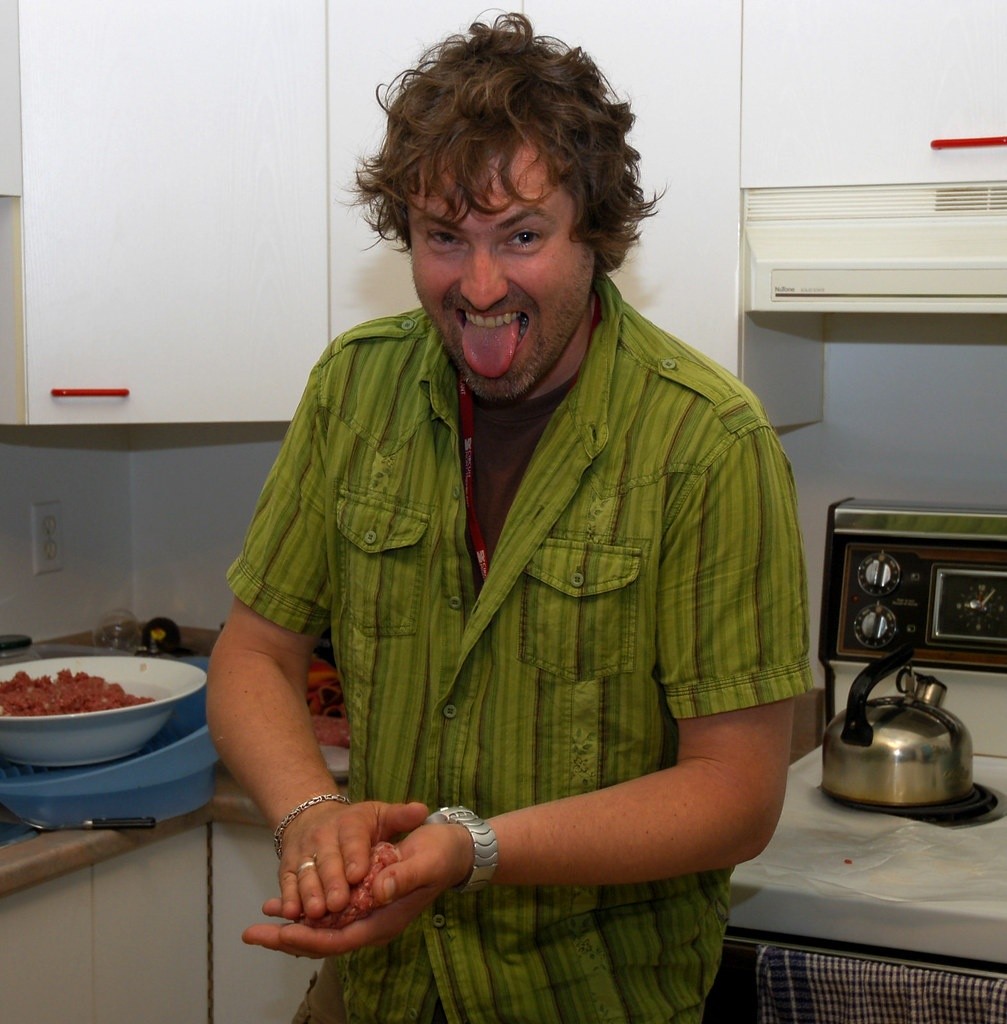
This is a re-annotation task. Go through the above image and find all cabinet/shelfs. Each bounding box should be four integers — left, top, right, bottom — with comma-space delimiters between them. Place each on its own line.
0, 820, 323, 1024
0, 0, 1007, 428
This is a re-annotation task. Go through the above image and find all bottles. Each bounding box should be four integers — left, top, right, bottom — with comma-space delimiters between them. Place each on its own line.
0, 634, 32, 665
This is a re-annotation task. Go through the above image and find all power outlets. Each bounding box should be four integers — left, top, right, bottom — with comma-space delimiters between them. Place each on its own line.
31, 500, 60, 575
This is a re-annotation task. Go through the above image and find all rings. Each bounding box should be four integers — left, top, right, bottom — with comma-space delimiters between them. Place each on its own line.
296, 863, 315, 874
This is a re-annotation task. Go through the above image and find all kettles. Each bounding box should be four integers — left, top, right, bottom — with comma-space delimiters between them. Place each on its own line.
823, 647, 973, 805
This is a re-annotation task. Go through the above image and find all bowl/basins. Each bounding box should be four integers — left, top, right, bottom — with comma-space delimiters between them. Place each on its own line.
0, 655, 207, 766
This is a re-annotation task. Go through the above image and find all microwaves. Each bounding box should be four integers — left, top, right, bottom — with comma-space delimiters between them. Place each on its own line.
819, 496, 1007, 676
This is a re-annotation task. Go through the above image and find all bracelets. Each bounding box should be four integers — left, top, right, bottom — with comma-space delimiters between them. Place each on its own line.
270, 793, 352, 861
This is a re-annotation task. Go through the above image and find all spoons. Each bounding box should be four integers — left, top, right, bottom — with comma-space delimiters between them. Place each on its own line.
20, 816, 156, 830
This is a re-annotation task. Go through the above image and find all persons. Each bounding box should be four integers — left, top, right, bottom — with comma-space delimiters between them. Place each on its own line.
205, 12, 810, 1024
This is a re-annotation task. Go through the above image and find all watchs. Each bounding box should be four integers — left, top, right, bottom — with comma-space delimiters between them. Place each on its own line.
422, 805, 498, 894
